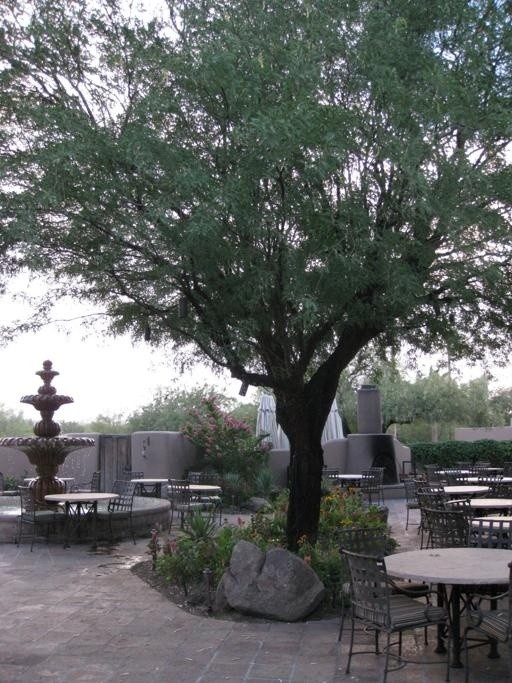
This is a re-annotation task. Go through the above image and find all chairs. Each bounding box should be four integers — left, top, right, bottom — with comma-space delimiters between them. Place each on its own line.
325, 457, 512, 530
337, 546, 451, 681
335, 528, 431, 646
15, 469, 225, 554
420, 507, 472, 547
462, 561, 512, 682
